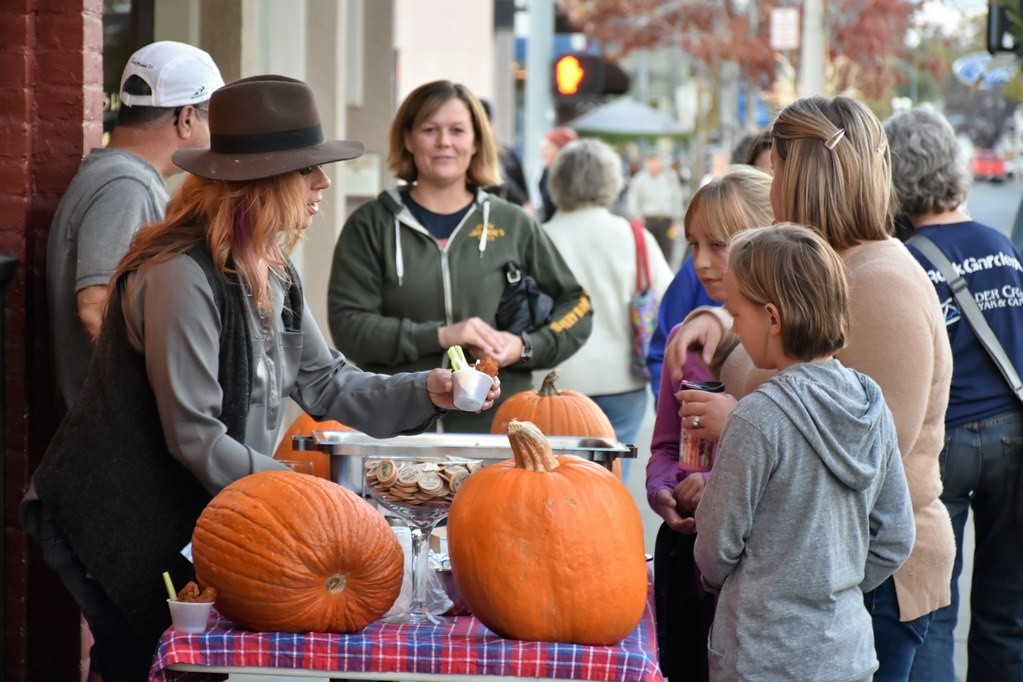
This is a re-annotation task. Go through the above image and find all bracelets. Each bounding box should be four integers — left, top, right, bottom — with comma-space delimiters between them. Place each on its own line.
519, 331, 532, 364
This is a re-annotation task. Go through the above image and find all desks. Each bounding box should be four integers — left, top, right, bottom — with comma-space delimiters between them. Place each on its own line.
148, 554, 669, 682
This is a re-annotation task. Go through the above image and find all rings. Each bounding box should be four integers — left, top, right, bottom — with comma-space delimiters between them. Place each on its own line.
693, 417, 699, 427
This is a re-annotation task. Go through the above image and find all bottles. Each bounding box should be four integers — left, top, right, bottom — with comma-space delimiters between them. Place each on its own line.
379, 517, 413, 618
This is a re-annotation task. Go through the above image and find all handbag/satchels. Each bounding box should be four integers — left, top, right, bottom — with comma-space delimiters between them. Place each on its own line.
495, 262, 553, 336
631, 216, 677, 377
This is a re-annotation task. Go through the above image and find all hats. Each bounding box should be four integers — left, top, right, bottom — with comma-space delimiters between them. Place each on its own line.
114, 41, 225, 105
170, 74, 365, 181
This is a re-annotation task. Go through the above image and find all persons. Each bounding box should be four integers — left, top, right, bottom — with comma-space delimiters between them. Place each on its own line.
540, 141, 676, 487
646, 170, 774, 682
883, 108, 1023, 682
693, 222, 916, 682
665, 96, 956, 682
19, 75, 501, 682
47, 40, 226, 682
327, 79, 595, 432
478, 98, 577, 220
646, 135, 773, 413
625, 152, 734, 266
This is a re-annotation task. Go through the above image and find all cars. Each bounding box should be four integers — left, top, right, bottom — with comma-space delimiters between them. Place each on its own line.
970, 149, 1005, 184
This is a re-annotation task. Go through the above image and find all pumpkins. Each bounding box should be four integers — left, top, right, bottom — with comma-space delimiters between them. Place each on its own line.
447, 418, 649, 647
491, 370, 622, 481
191, 471, 406, 634
271, 410, 358, 481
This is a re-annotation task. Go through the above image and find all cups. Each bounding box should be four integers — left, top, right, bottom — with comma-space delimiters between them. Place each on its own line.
166, 599, 215, 634
451, 369, 495, 413
678, 381, 726, 472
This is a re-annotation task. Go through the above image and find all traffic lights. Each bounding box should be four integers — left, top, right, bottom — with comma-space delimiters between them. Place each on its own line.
548, 52, 602, 100
